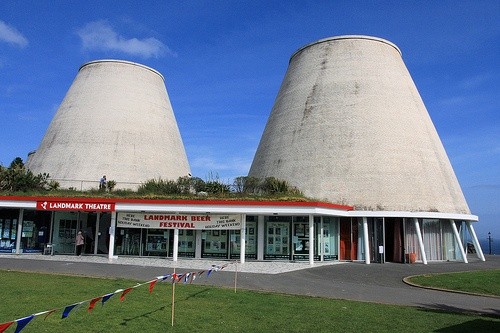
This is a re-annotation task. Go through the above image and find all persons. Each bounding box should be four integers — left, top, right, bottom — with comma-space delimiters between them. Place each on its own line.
76, 231, 84, 256
99, 176, 107, 191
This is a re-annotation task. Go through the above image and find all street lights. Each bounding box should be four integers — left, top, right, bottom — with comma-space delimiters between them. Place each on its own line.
488, 232, 493, 254
